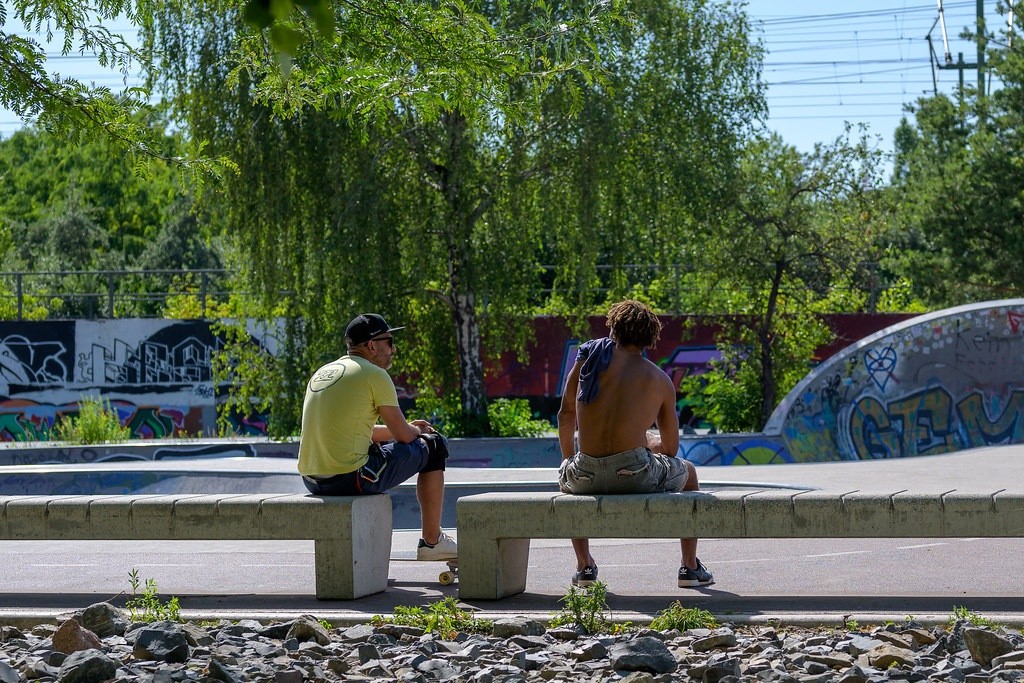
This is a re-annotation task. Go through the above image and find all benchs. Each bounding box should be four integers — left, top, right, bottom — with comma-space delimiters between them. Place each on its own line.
454, 484, 1024, 608
0, 488, 394, 606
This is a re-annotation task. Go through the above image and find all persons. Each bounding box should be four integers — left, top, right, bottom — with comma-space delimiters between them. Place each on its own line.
298, 313, 457, 561
557, 300, 714, 587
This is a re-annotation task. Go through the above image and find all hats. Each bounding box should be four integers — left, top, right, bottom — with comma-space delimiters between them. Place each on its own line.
345, 313, 406, 347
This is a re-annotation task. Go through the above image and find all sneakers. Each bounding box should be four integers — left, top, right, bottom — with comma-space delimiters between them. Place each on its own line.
416, 533, 457, 561
678, 557, 714, 587
572, 566, 598, 586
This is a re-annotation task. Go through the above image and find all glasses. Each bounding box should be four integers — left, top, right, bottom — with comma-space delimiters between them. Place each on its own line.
364, 336, 394, 348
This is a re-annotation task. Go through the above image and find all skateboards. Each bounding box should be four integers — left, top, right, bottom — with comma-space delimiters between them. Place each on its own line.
389, 556, 457, 586
